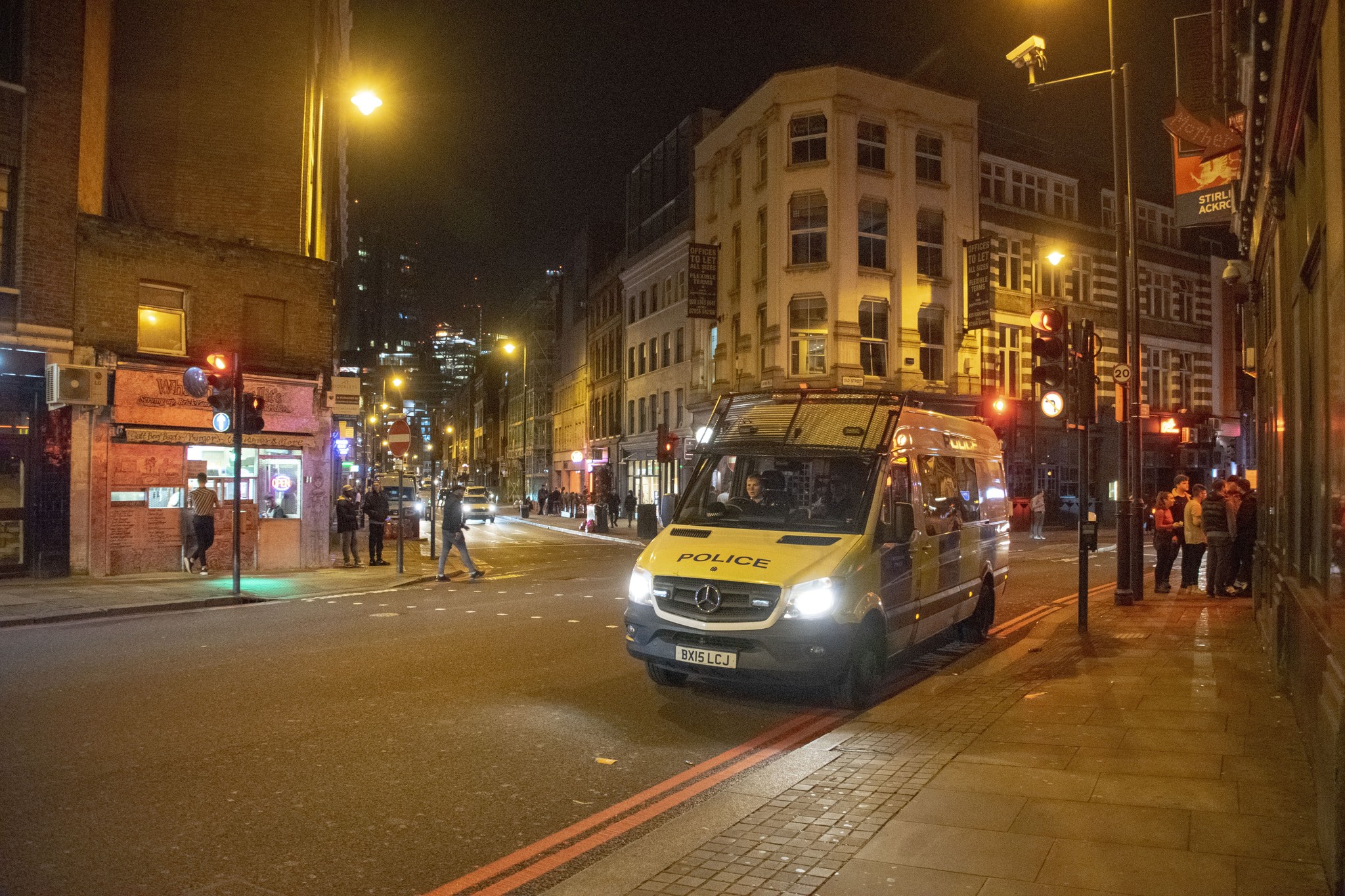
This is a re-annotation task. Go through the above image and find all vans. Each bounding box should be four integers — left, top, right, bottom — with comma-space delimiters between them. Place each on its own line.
624, 389, 1010, 711
374, 472, 432, 538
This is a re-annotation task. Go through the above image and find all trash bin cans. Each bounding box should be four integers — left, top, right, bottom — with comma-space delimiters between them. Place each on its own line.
520, 505, 530, 518
402, 506, 421, 538
636, 504, 658, 539
587, 502, 610, 532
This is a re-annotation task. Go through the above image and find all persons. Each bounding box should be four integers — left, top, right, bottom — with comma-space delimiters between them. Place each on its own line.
166, 473, 220, 576
435, 485, 484, 581
259, 493, 284, 518
336, 479, 390, 567
1029, 489, 1047, 540
708, 473, 848, 517
280, 481, 298, 515
519, 484, 636, 529
1146, 474, 1256, 597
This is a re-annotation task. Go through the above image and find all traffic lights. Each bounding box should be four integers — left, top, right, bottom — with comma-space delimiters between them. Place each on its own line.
244, 394, 264, 435
1030, 304, 1069, 434
663, 442, 673, 464
1067, 319, 1097, 425
205, 352, 235, 434
655, 423, 667, 464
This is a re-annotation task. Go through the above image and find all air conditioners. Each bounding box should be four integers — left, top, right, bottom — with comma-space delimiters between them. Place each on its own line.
46, 363, 108, 405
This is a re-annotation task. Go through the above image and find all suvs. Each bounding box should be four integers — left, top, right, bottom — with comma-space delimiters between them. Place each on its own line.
464, 486, 489, 499
462, 494, 495, 523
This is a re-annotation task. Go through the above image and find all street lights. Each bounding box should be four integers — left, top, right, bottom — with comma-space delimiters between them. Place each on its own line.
364, 393, 388, 486
382, 376, 401, 471
1028, 234, 1067, 513
504, 331, 526, 505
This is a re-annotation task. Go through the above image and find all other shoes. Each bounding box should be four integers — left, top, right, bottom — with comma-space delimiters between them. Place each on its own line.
548, 514, 560, 516
200, 568, 208, 575
184, 557, 194, 575
611, 526, 614, 528
343, 560, 354, 567
469, 570, 485, 580
369, 559, 377, 566
613, 521, 618, 527
354, 559, 369, 567
376, 559, 390, 566
1034, 535, 1046, 539
436, 574, 451, 582
1154, 581, 1238, 599
628, 524, 631, 527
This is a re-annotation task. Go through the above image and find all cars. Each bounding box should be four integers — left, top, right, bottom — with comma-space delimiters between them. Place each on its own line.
437, 487, 450, 500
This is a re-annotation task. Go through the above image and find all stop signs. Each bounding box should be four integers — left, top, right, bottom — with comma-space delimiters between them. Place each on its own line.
388, 420, 410, 457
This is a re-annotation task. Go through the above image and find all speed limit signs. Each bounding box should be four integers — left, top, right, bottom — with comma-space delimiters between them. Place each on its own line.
1112, 363, 1131, 383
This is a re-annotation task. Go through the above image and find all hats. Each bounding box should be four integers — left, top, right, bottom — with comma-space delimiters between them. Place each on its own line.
342, 484, 353, 492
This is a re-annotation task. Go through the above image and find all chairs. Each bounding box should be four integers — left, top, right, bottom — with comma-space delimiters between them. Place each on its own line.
761, 470, 793, 509
